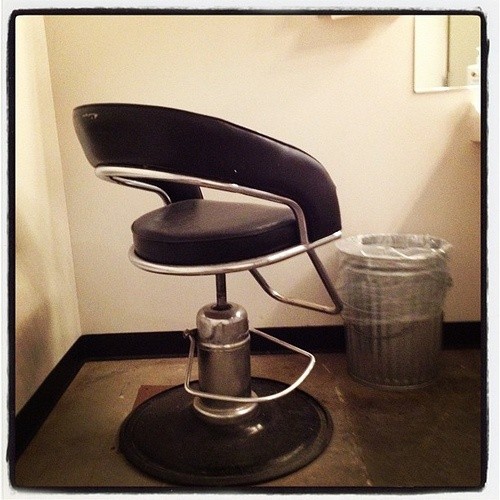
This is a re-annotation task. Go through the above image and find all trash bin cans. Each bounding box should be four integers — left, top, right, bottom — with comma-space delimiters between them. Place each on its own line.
335, 234, 453, 391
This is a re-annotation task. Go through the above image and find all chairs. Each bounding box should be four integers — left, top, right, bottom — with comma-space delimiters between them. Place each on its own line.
73, 103, 344, 487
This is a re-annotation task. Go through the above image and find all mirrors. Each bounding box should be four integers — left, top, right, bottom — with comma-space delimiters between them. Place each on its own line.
446, 16, 481, 86
